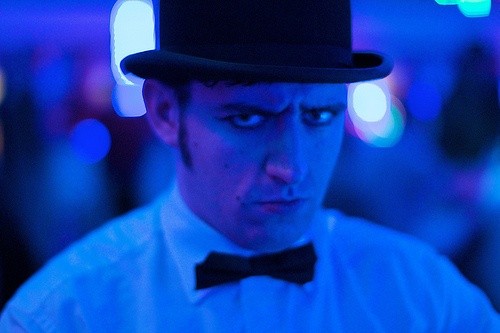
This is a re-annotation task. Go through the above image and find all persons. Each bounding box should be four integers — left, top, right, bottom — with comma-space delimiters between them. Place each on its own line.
1, 1, 500, 333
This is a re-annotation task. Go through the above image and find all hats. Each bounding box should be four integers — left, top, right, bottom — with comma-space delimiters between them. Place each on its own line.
119, 0, 394, 82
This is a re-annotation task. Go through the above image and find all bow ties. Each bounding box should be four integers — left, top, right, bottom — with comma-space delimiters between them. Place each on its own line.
194, 240, 317, 290
